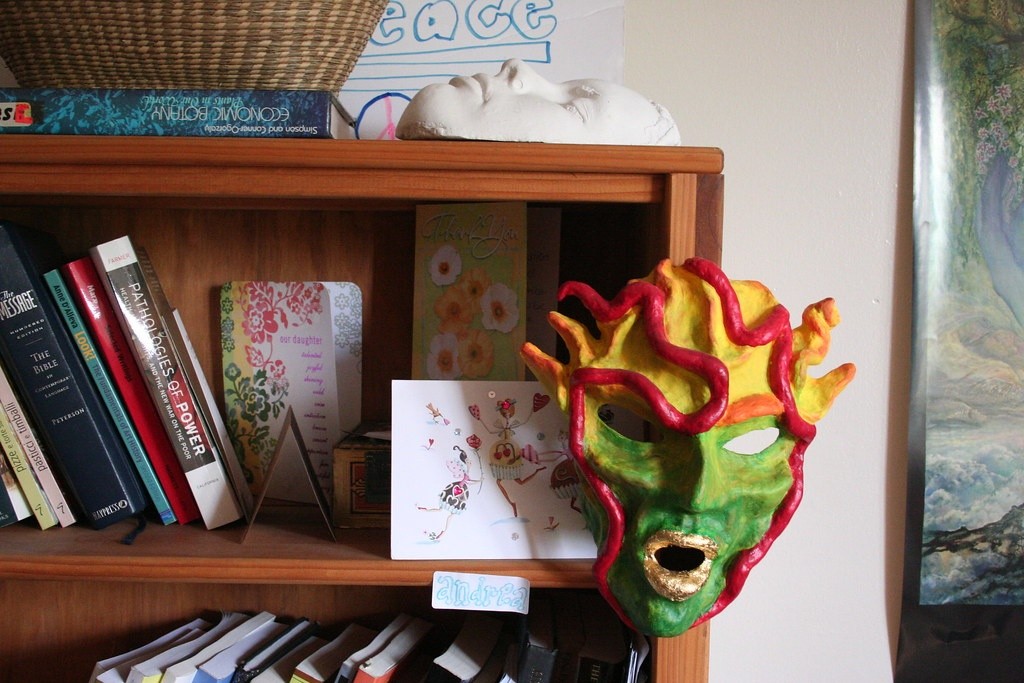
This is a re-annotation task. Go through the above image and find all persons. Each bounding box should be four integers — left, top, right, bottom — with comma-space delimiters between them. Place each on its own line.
394, 56, 682, 148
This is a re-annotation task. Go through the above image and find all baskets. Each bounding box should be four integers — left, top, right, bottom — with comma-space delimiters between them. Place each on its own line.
0, 0, 390, 93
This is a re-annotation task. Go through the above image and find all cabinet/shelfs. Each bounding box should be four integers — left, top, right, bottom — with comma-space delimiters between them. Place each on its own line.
0, 134, 726, 683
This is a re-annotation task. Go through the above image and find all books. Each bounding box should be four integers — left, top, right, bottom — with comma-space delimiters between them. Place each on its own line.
0, 200, 597, 561
0, 87, 358, 141
88, 589, 650, 683
